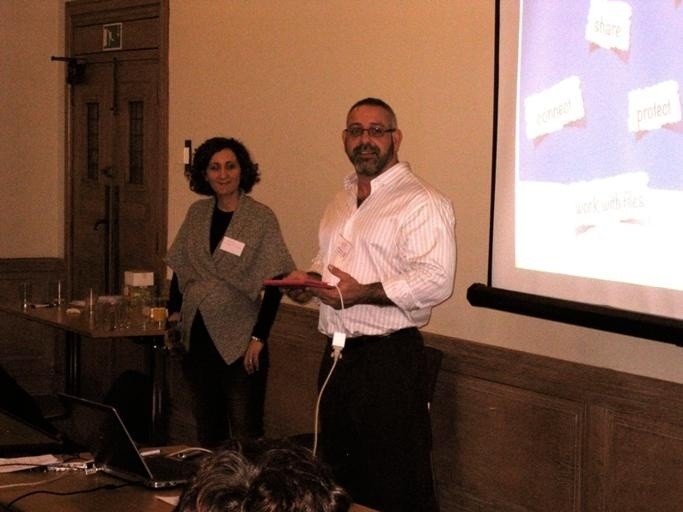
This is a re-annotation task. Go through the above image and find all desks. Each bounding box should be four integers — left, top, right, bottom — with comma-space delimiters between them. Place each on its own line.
0, 466, 376, 512
0, 295, 173, 424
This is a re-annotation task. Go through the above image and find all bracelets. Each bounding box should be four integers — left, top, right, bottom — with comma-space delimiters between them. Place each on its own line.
250, 336, 264, 343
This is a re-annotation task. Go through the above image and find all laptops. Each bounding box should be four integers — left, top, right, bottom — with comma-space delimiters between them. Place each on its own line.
57, 391, 200, 490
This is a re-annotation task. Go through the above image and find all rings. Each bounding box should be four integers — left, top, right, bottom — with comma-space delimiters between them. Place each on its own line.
248, 359, 251, 364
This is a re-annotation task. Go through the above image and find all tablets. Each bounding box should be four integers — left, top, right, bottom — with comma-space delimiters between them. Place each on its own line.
263, 279, 332, 288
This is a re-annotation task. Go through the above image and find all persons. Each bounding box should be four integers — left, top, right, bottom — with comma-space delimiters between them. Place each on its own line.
171, 435, 353, 512
167, 137, 297, 454
281, 97, 457, 512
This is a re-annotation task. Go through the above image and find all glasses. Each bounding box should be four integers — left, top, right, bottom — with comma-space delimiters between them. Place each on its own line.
345, 126, 396, 137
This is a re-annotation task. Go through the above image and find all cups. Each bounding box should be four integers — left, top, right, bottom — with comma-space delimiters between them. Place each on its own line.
47, 279, 68, 305
151, 308, 166, 329
84, 288, 99, 332
18, 281, 35, 310
